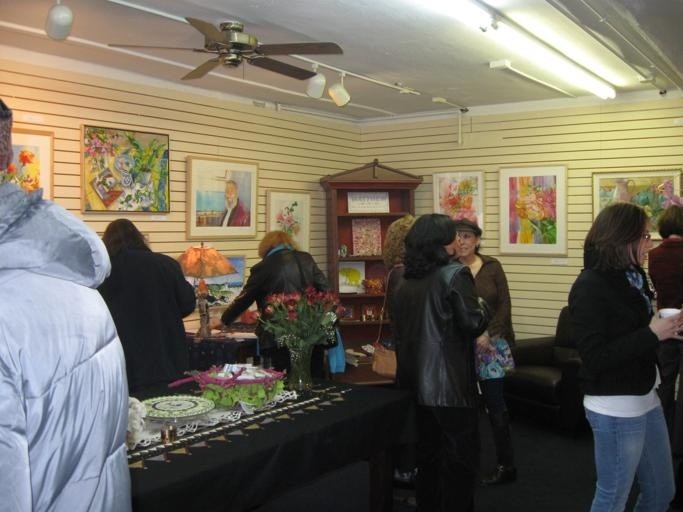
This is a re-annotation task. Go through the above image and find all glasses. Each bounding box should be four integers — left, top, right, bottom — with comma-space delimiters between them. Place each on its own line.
642, 234, 651, 240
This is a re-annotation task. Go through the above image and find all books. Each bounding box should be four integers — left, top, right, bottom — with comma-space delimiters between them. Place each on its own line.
341, 345, 377, 370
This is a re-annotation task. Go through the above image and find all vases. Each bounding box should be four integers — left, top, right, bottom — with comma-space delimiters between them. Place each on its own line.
285, 343, 316, 391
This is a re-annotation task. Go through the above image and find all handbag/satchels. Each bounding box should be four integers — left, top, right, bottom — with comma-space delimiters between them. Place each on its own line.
327, 327, 346, 374
372, 346, 398, 378
477, 336, 516, 381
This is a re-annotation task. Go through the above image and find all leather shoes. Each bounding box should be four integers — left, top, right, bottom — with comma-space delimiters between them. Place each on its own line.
482, 466, 516, 484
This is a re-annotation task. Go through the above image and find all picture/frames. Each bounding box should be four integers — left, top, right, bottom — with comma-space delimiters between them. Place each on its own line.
76, 123, 312, 269
431, 164, 486, 240
2, 128, 54, 204
497, 164, 567, 255
591, 170, 682, 241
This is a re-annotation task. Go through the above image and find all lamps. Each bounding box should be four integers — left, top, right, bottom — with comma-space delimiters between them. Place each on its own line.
44, 0, 73, 42
304, 64, 351, 108
174, 240, 238, 338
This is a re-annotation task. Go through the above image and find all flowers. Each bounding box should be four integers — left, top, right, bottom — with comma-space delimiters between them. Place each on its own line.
257, 287, 343, 386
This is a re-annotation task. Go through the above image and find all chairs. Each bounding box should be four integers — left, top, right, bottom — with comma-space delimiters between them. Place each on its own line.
506, 304, 584, 431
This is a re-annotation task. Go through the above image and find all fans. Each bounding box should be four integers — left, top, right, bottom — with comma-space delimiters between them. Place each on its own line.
105, 17, 343, 81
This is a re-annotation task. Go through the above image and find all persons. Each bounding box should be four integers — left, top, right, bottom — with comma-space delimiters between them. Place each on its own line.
381, 214, 426, 488
215, 180, 252, 227
450, 220, 521, 487
1, 97, 131, 512
552, 203, 682, 512
647, 206, 682, 416
209, 230, 334, 375
98, 218, 195, 390
383, 214, 490, 511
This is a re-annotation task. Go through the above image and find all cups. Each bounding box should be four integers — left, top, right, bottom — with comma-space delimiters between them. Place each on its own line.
655, 308, 679, 332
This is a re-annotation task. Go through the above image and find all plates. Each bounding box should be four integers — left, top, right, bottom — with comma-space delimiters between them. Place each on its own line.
139, 393, 215, 420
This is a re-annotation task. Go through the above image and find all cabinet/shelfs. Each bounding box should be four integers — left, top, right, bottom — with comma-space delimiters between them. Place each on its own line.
320, 159, 425, 361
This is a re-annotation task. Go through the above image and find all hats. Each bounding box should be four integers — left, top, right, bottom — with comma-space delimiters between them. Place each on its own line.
454, 220, 481, 236
